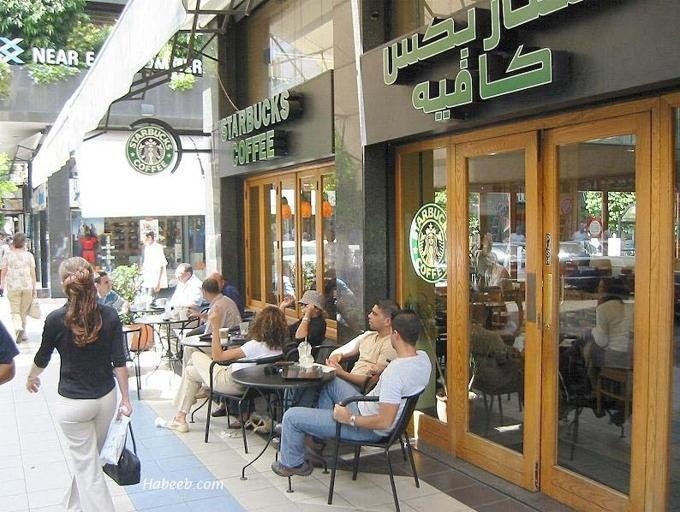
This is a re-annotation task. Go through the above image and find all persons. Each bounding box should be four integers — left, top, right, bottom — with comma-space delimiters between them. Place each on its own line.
24, 253, 133, 512
571, 222, 587, 242
270, 308, 434, 479
508, 221, 524, 242
270, 299, 405, 451
468, 295, 524, 400
577, 275, 633, 402
0, 229, 329, 434
476, 234, 499, 290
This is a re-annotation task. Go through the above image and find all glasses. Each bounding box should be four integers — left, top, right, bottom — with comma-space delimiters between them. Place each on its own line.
299, 303, 309, 308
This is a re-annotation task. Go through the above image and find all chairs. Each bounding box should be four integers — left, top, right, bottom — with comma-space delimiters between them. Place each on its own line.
471, 350, 523, 425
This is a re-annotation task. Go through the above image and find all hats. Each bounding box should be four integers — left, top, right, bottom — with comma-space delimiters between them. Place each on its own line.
296, 290, 326, 312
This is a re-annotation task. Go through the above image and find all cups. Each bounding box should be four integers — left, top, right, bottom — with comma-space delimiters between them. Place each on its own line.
297, 341, 315, 369
239, 321, 249, 335
136, 296, 144, 310
160, 298, 167, 306
179, 309, 189, 321
218, 327, 229, 345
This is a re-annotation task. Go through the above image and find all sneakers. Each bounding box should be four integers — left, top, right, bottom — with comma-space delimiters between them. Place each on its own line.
271, 461, 314, 478
212, 407, 227, 417
231, 420, 244, 427
166, 416, 189, 433
16, 327, 25, 344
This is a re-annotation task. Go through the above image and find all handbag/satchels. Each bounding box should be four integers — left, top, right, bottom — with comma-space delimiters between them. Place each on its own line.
103, 413, 142, 487
243, 414, 277, 435
29, 297, 41, 319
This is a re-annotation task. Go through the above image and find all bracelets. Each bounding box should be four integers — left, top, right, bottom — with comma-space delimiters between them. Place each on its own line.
349, 413, 356, 427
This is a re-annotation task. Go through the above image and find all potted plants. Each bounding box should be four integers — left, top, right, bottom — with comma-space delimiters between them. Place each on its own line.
109, 262, 155, 352
